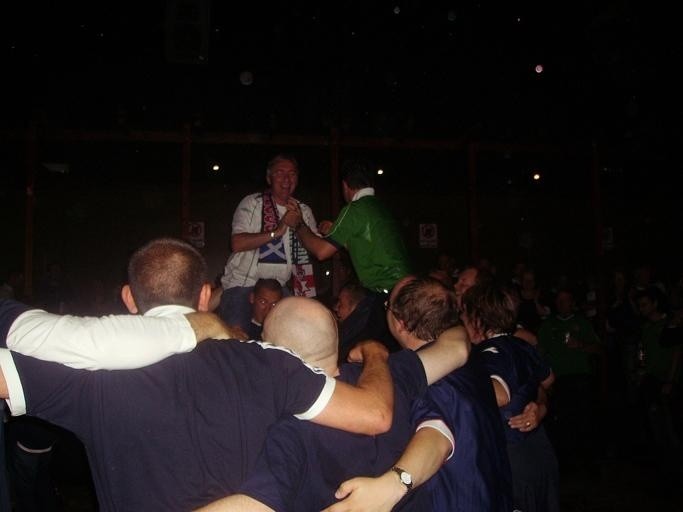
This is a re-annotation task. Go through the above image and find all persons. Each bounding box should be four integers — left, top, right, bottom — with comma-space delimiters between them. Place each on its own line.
0, 153, 682, 510
